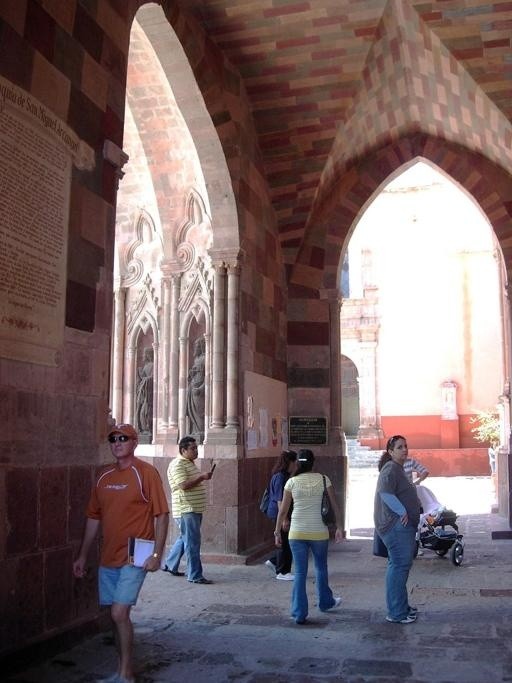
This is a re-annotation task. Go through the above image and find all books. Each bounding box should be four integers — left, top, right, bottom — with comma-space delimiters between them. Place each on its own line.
128, 535, 157, 565
133, 537, 155, 567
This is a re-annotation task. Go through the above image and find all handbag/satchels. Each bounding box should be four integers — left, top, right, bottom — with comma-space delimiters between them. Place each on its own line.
373, 529, 388, 558
321, 491, 336, 526
259, 488, 270, 514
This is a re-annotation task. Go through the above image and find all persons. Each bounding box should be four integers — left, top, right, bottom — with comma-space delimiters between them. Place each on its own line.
71, 422, 170, 682
398, 456, 430, 489
158, 435, 215, 584
273, 447, 344, 624
371, 435, 422, 624
263, 448, 299, 581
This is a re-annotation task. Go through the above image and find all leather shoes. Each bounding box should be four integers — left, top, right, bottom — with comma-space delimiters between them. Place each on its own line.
190, 578, 213, 583
162, 565, 185, 576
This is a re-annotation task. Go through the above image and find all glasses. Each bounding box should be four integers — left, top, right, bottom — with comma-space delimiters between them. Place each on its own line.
108, 434, 130, 443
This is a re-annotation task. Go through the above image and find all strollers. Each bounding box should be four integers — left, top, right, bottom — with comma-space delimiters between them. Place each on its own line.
410, 483, 461, 534
416, 516, 470, 565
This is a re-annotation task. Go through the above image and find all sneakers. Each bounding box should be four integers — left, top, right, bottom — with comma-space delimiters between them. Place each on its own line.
332, 598, 342, 608
265, 559, 276, 572
276, 573, 295, 581
386, 607, 417, 623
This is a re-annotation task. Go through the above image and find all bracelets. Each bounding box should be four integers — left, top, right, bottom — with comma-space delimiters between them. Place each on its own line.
274, 531, 281, 536
151, 552, 161, 560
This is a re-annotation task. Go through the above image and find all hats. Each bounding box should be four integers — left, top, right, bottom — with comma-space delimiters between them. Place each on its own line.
108, 424, 137, 439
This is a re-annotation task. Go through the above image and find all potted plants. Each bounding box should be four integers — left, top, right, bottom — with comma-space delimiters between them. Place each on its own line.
469, 408, 505, 473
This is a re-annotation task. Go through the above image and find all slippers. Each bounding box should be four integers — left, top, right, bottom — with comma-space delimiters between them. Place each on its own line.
96, 671, 135, 683
